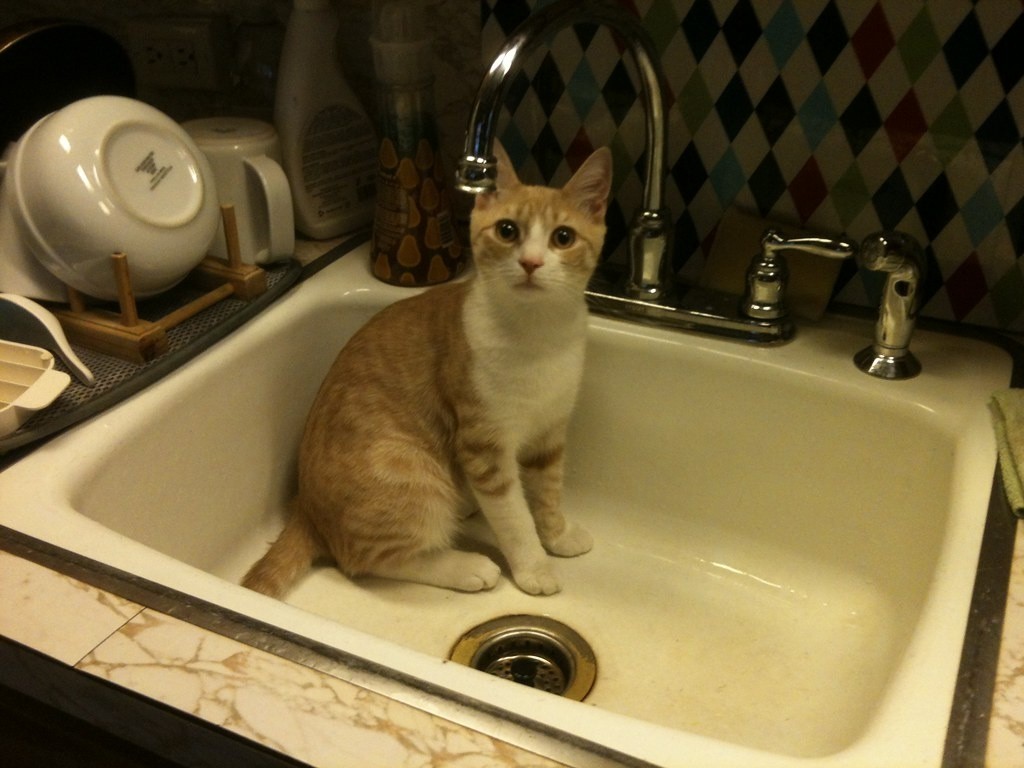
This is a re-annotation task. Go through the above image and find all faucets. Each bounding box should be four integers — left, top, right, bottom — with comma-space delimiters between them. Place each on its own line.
449, 8, 673, 323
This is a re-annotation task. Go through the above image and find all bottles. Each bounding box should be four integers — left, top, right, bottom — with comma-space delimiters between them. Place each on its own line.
367, 2, 468, 289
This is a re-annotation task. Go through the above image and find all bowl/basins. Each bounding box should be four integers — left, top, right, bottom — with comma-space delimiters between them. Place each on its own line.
0, 19, 135, 167
5, 97, 220, 302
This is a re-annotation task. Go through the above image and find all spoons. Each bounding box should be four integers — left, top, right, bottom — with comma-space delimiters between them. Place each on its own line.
0, 293, 95, 389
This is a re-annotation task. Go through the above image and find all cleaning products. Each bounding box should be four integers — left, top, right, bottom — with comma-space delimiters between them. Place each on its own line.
359, 3, 470, 288
268, 2, 379, 240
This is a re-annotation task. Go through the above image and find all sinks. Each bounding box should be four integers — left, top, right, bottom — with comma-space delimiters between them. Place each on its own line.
0, 234, 1016, 766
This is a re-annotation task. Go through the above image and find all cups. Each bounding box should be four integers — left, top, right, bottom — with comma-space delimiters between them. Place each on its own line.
178, 114, 296, 267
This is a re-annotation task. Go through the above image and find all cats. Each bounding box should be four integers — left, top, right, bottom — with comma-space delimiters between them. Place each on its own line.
240, 138, 612, 603
